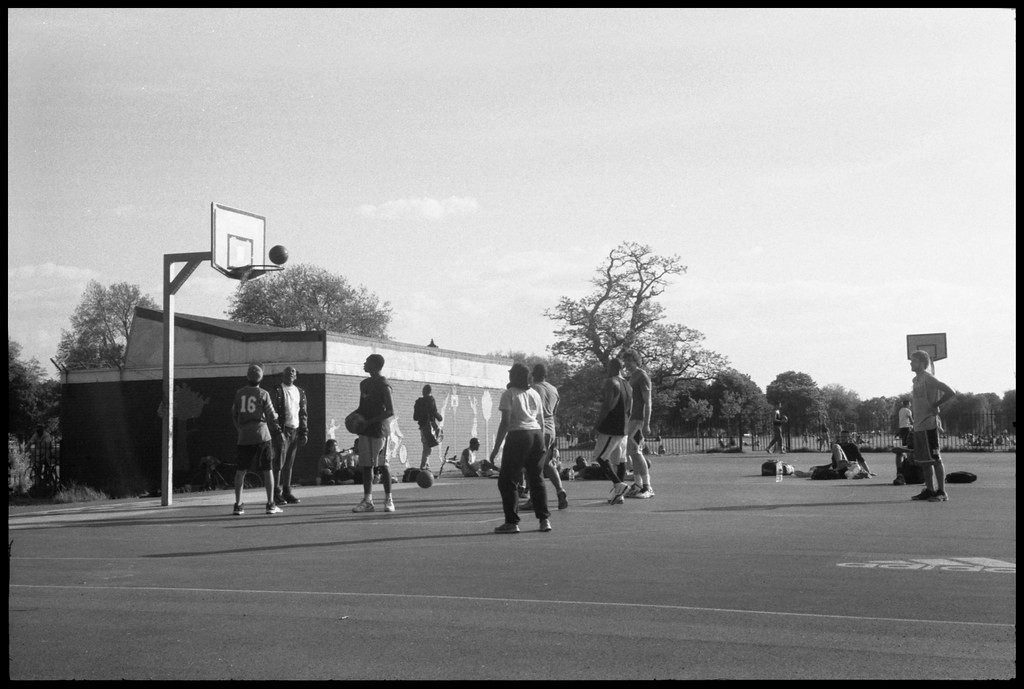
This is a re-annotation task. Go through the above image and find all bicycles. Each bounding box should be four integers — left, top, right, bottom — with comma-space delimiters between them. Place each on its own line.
21, 451, 72, 502
438, 446, 462, 476
189, 455, 263, 492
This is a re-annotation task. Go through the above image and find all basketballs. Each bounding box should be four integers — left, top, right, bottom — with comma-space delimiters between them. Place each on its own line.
268, 245, 289, 264
416, 470, 434, 489
345, 413, 366, 433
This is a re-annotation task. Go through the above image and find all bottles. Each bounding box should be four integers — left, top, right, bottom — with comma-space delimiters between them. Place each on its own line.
776, 462, 783, 483
569, 467, 574, 483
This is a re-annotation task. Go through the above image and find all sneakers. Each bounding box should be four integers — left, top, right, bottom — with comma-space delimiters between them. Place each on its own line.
911, 489, 934, 500
636, 487, 655, 499
352, 499, 376, 512
265, 503, 283, 513
383, 498, 395, 511
624, 483, 642, 497
608, 483, 629, 504
539, 519, 552, 531
494, 523, 520, 533
929, 490, 948, 502
519, 500, 535, 510
233, 503, 245, 514
557, 490, 568, 509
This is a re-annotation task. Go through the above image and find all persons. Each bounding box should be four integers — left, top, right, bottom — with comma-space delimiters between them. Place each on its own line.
230, 361, 285, 515
318, 438, 398, 485
460, 438, 500, 477
25, 423, 54, 482
764, 400, 786, 454
588, 358, 633, 505
573, 456, 608, 479
719, 435, 735, 447
801, 424, 867, 450
831, 431, 876, 476
899, 401, 913, 446
413, 384, 442, 469
344, 354, 396, 512
490, 363, 570, 534
269, 366, 309, 505
620, 348, 654, 498
959, 432, 1008, 445
909, 350, 954, 502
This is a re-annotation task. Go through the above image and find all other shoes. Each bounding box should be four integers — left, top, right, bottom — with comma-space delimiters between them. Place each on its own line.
282, 487, 300, 503
275, 487, 287, 505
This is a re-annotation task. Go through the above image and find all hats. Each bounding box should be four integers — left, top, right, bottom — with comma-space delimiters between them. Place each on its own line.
246, 361, 264, 383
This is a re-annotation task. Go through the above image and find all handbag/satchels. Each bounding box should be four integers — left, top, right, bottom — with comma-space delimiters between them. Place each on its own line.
423, 420, 444, 447
